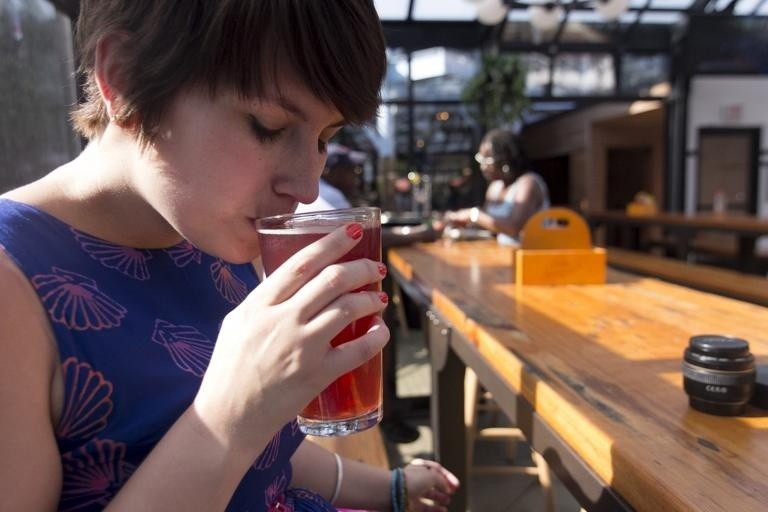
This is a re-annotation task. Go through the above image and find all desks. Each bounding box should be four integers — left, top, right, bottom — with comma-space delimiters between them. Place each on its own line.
383, 209, 766, 512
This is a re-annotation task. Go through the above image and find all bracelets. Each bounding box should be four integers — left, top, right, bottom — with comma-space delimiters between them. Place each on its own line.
401, 225, 411, 246
329, 452, 343, 505
390, 467, 409, 512
466, 206, 480, 228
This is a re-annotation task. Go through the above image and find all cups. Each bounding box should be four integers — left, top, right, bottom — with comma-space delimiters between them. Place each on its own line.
252, 205, 384, 441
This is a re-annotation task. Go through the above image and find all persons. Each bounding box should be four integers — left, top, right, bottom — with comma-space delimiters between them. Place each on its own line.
0, 0, 463, 512
291, 144, 445, 445
431, 128, 552, 248
